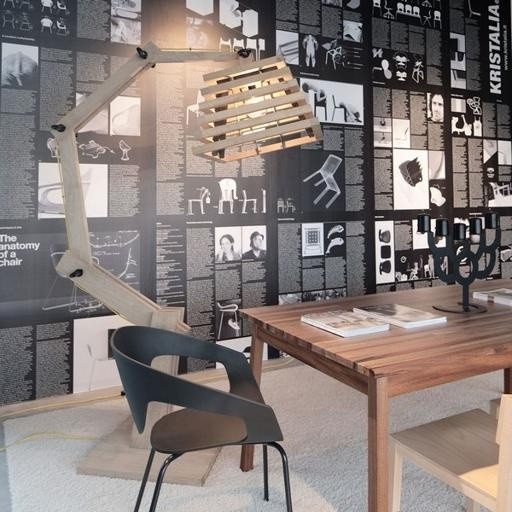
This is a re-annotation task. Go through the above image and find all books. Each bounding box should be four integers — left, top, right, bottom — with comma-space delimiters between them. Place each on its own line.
301, 309, 389, 337
353, 303, 447, 329
473, 288, 512, 306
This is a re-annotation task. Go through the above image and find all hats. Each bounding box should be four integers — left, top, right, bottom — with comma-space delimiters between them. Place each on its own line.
250, 231, 264, 240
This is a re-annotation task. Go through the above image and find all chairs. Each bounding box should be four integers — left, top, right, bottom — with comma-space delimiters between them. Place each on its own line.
390, 392, 511, 512
110, 325, 295, 512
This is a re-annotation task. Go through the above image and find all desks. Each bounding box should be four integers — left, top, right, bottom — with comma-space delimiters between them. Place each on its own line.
236, 272, 511, 511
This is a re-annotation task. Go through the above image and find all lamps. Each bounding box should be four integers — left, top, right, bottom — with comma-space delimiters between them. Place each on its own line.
46, 40, 326, 488
416, 207, 503, 316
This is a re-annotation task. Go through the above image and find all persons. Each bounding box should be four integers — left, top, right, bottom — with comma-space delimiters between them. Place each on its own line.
242, 231, 266, 259
427, 93, 444, 123
215, 234, 241, 261
